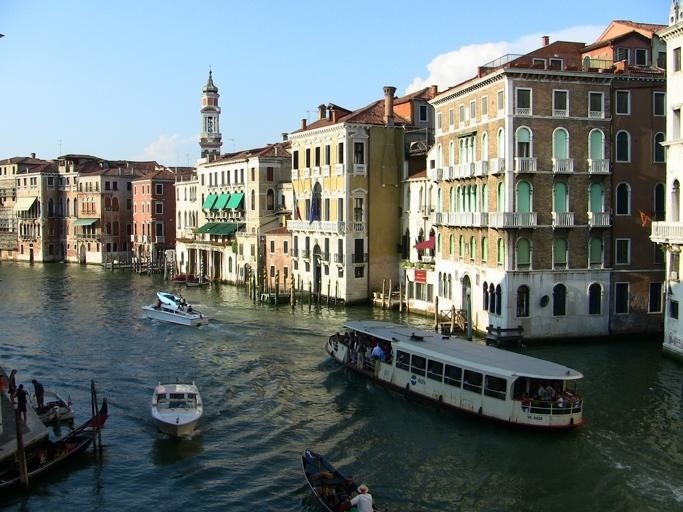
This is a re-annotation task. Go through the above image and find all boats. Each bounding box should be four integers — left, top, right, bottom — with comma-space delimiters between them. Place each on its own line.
151, 381, 203, 437
142, 292, 208, 327
325, 321, 583, 427
301, 448, 378, 512
0, 388, 109, 488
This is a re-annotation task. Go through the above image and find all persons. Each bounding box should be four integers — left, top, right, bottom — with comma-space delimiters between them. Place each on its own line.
513, 382, 580, 413
12, 384, 28, 423
187, 305, 192, 313
31, 379, 43, 408
178, 297, 185, 309
332, 330, 392, 364
337, 484, 374, 511
7, 369, 17, 402
154, 300, 161, 310
340, 477, 357, 500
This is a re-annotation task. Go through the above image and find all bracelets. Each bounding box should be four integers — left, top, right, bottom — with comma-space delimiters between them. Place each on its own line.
346, 496, 349, 498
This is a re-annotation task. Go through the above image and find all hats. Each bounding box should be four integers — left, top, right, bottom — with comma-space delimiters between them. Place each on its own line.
357, 484, 369, 494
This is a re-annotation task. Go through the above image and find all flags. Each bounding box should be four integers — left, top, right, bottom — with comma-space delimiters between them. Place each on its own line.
638, 209, 649, 228
309, 191, 316, 227
